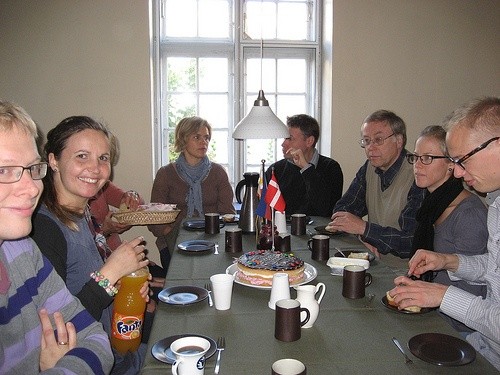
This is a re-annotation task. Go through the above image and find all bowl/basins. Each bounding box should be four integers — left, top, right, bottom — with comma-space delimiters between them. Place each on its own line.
327, 257, 369, 276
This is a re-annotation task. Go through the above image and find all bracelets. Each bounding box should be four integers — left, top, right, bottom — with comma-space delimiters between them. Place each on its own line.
98, 223, 113, 237
95, 269, 119, 294
90, 271, 114, 297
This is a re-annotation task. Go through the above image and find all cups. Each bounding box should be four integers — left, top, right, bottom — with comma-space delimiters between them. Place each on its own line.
342, 265, 372, 298
170, 337, 211, 375
277, 233, 291, 252
271, 358, 306, 375
291, 214, 307, 236
211, 273, 234, 311
205, 212, 225, 234
224, 228, 243, 253
268, 273, 311, 342
307, 234, 330, 261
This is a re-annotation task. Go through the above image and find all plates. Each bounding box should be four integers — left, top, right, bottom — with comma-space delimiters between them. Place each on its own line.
158, 285, 209, 305
225, 263, 317, 289
177, 239, 215, 253
407, 333, 476, 367
334, 250, 375, 263
152, 334, 217, 363
314, 225, 344, 234
381, 295, 438, 314
183, 220, 204, 230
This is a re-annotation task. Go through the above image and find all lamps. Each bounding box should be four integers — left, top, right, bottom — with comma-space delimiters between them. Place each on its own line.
233, 0, 291, 141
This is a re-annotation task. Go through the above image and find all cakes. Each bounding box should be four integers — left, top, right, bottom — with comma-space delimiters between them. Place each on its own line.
237, 250, 305, 286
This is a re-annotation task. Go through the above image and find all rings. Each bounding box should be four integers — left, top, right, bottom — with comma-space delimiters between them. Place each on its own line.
140, 253, 144, 259
58, 342, 67, 345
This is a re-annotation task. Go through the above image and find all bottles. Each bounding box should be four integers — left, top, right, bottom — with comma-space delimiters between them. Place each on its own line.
111, 260, 149, 355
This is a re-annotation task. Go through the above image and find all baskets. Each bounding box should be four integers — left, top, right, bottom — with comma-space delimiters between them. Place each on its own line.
109, 209, 182, 226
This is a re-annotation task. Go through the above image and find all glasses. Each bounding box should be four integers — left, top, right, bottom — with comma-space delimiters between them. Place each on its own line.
405, 153, 452, 165
444, 136, 500, 171
0, 162, 49, 184
360, 133, 394, 146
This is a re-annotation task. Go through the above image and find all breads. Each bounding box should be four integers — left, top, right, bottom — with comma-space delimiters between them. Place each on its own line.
223, 214, 235, 222
386, 291, 421, 312
325, 225, 338, 232
120, 202, 140, 213
349, 252, 369, 259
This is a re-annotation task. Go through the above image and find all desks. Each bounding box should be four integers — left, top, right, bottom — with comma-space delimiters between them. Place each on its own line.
142, 214, 500, 375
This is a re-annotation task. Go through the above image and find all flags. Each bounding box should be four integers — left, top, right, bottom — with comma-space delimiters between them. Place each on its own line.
256, 163, 284, 222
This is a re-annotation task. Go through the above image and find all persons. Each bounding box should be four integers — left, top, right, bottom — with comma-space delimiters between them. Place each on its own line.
407, 124, 488, 336
148, 117, 233, 269
0, 101, 115, 375
29, 115, 167, 375
325, 109, 426, 278
266, 115, 343, 218
389, 94, 500, 370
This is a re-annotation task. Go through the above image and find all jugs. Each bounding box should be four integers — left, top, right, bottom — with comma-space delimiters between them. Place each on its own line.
294, 283, 325, 329
235, 172, 261, 233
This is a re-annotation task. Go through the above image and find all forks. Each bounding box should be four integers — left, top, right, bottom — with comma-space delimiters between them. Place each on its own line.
213, 337, 225, 374
392, 337, 415, 367
214, 241, 219, 254
204, 283, 213, 306
